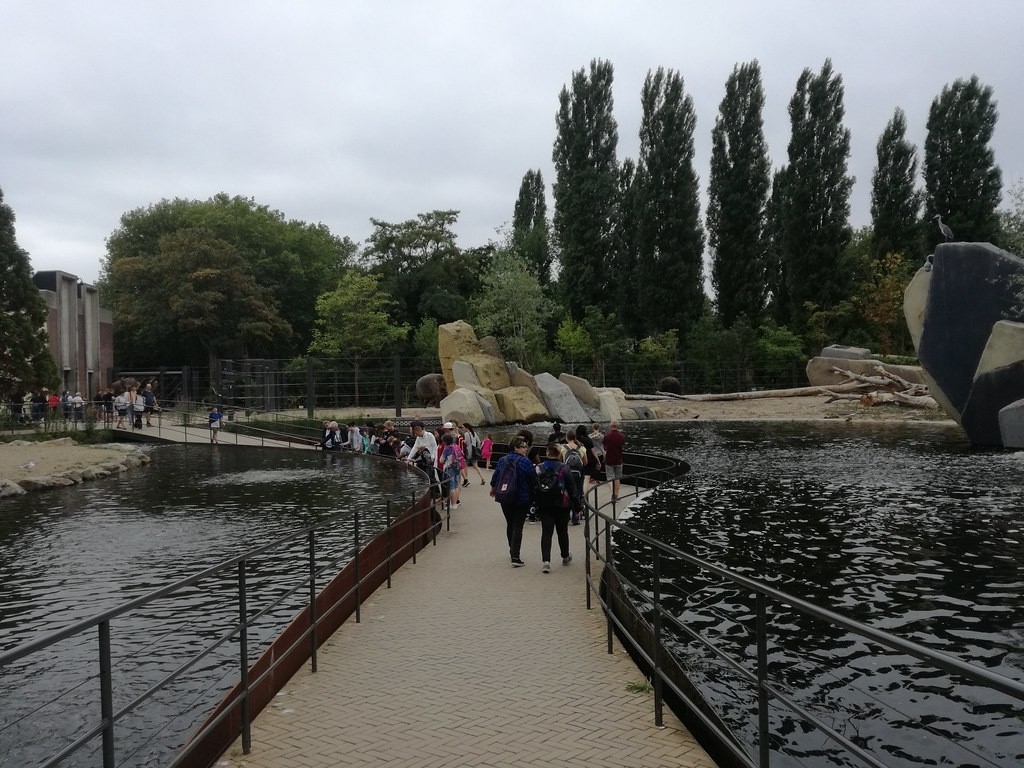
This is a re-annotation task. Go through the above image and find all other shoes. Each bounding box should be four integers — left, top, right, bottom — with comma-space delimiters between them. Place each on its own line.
590, 478, 594, 485
612, 495, 621, 503
543, 564, 551, 572
462, 479, 470, 486
436, 499, 445, 504
511, 558, 525, 566
530, 518, 537, 524
444, 504, 458, 509
573, 521, 580, 525
563, 553, 572, 565
480, 479, 485, 485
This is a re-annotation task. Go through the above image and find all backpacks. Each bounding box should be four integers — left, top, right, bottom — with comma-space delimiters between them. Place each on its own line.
563, 443, 583, 478
494, 456, 523, 503
399, 436, 416, 452
535, 462, 565, 496
455, 433, 466, 451
447, 444, 466, 471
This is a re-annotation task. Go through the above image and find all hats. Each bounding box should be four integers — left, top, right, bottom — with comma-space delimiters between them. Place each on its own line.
443, 422, 453, 428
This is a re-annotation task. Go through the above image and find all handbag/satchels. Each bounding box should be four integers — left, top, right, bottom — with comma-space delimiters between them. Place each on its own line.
472, 445, 482, 460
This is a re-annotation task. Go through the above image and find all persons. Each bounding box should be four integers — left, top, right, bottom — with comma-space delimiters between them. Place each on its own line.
208, 407, 224, 443
20, 381, 158, 430
314, 419, 626, 572
